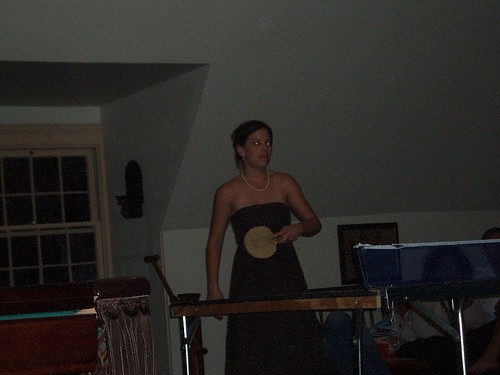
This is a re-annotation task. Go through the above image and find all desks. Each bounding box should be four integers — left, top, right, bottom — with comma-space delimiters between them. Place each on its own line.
171, 277, 500, 375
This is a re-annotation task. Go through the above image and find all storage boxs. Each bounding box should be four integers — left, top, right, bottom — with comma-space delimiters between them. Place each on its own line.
353, 238, 500, 289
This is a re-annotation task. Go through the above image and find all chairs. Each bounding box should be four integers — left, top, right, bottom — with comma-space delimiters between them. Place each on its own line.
371, 308, 432, 375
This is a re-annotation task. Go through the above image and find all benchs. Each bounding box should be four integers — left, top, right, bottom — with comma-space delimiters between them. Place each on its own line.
0, 277, 152, 375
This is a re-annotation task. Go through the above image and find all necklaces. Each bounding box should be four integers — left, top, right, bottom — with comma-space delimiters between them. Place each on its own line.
241, 168, 270, 192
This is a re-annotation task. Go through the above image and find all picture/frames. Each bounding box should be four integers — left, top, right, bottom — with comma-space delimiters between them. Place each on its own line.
337, 222, 402, 285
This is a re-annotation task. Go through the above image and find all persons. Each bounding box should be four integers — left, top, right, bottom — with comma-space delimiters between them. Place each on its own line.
393, 227, 500, 375
206, 120, 331, 375
